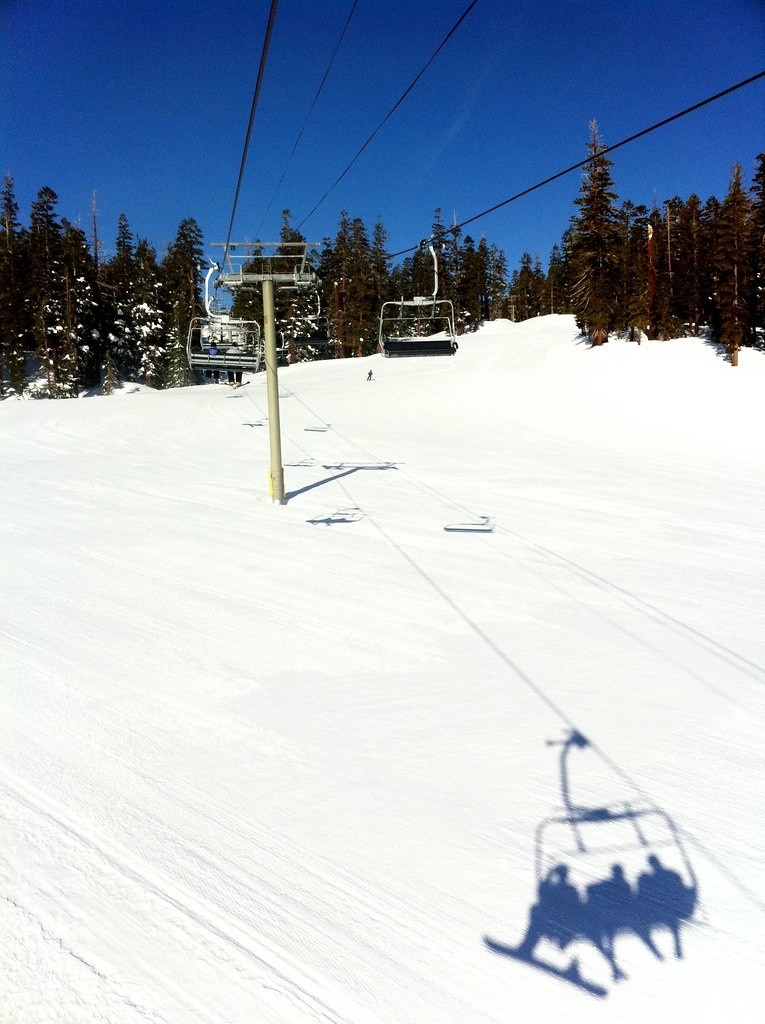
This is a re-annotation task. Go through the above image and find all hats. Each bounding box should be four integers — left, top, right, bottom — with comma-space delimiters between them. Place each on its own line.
211, 342, 216, 348
232, 340, 238, 347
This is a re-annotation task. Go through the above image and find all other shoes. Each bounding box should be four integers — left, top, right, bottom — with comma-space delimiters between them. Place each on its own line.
215, 375, 219, 379
229, 381, 235, 385
236, 382, 242, 386
206, 376, 210, 381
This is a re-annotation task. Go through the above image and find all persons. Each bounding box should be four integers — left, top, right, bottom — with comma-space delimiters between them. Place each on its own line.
367, 370, 372, 381
205, 342, 222, 379
225, 342, 242, 385
